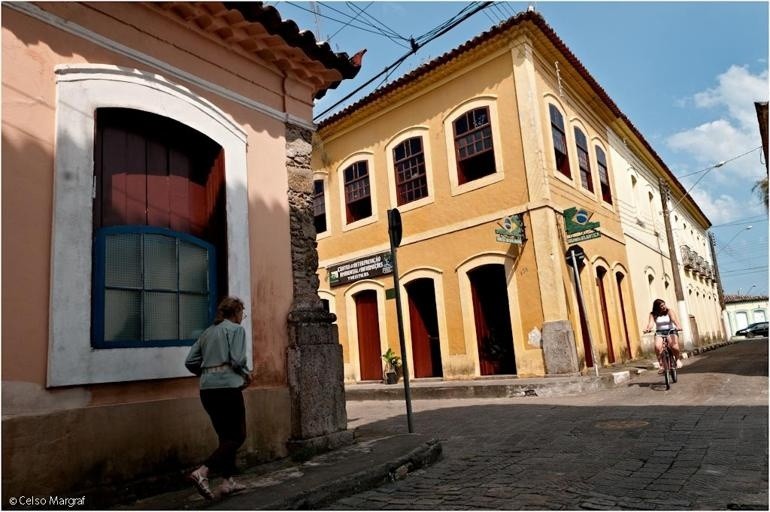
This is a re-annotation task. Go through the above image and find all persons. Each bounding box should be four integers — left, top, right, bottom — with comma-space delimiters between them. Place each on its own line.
642, 298, 681, 374
185, 297, 253, 500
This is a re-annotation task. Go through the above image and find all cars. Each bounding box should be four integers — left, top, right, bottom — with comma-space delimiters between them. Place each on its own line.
736, 322, 769, 338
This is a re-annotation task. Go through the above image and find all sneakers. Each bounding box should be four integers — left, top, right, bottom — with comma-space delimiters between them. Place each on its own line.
676, 361, 683, 369
658, 367, 665, 374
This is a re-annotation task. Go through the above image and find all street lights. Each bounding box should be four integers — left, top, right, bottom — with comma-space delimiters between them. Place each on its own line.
707, 226, 753, 341
658, 161, 727, 351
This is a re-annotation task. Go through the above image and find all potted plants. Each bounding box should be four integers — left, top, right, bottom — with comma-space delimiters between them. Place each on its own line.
381, 348, 401, 384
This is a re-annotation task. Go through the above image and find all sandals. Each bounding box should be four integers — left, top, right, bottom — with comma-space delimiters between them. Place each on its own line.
220, 481, 247, 496
189, 470, 214, 501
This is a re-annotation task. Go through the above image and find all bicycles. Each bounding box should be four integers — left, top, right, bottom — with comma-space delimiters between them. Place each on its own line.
643, 328, 683, 390
